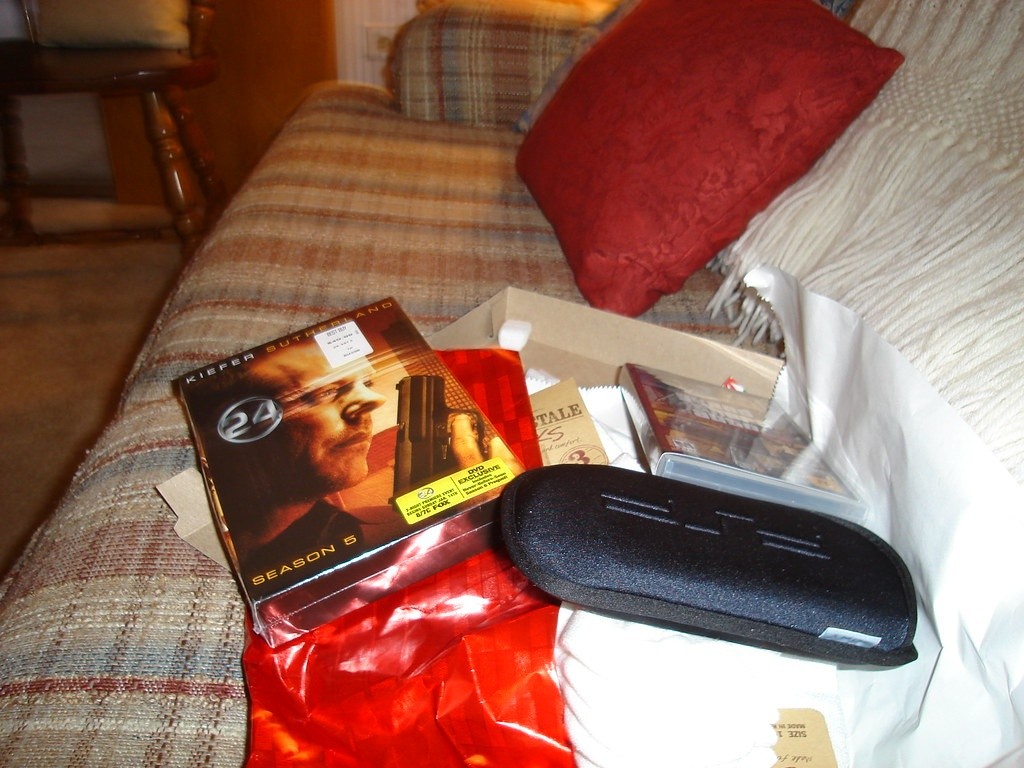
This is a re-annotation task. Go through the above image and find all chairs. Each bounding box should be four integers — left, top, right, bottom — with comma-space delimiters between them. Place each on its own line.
0, 0, 227, 261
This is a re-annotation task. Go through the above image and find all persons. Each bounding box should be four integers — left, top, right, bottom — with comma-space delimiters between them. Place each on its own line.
203, 337, 520, 605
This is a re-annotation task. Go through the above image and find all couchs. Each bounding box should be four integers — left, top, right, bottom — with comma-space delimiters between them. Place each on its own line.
0, 0, 1024, 768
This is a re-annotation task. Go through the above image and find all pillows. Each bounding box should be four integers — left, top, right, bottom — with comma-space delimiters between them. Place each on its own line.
516, 0, 906, 320
22, 0, 194, 49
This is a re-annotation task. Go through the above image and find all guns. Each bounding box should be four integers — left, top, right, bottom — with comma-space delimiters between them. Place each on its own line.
389, 374, 488, 514
